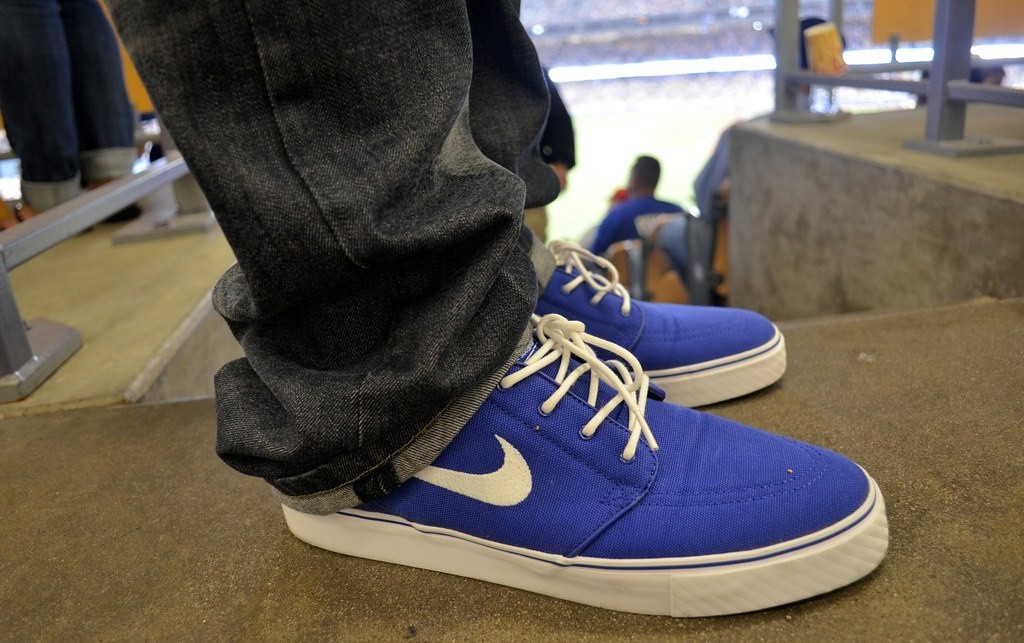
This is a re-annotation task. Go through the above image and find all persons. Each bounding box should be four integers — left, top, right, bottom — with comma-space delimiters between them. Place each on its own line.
103, 1, 890, 620
0, 2, 145, 240
653, 16, 1006, 301
575, 155, 692, 268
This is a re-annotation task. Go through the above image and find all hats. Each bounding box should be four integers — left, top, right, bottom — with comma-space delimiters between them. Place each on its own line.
768, 17, 846, 68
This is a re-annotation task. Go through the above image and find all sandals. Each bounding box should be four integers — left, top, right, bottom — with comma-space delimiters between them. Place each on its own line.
0, 199, 92, 236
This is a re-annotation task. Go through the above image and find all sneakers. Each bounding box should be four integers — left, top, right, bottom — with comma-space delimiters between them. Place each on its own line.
531, 232, 789, 407
276, 313, 894, 621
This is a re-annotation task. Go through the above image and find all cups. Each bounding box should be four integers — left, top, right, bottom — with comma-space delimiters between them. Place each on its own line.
803, 22, 850, 77
